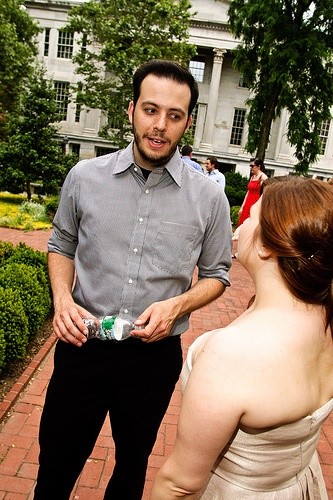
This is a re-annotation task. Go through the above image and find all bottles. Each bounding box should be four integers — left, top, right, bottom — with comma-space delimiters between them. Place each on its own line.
72, 315, 144, 341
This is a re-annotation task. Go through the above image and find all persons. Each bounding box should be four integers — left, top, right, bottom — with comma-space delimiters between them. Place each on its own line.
149, 175, 333, 500
202, 156, 225, 193
237, 157, 269, 228
32, 59, 232, 500
180, 145, 203, 174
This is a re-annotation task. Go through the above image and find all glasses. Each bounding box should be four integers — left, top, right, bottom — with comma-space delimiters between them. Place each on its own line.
249, 165, 256, 168
